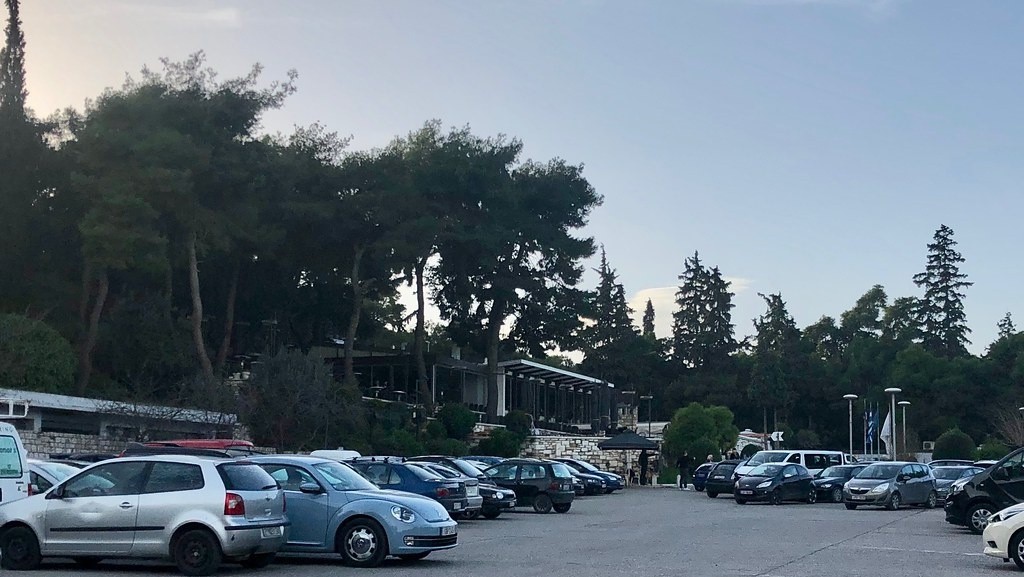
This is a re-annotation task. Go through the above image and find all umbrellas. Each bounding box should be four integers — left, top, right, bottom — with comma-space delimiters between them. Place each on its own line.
596, 429, 660, 450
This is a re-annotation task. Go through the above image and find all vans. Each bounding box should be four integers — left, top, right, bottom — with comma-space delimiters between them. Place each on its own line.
736, 449, 844, 481
0, 422, 30, 507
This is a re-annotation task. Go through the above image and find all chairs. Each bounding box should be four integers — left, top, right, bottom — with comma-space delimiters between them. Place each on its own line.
85, 471, 393, 496
880, 468, 898, 478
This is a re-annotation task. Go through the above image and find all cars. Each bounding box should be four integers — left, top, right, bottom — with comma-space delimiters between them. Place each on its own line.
483, 460, 574, 513
734, 461, 813, 506
551, 457, 624, 495
844, 454, 859, 464
691, 462, 718, 492
317, 448, 515, 521
0, 452, 288, 572
705, 459, 742, 498
926, 459, 1000, 507
844, 459, 938, 510
240, 455, 457, 565
814, 465, 870, 500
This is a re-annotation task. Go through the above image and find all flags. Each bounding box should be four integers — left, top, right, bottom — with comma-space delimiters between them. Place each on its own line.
866, 412, 878, 443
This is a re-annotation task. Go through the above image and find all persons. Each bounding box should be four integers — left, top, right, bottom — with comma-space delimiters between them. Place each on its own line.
705, 448, 740, 463
674, 450, 696, 491
638, 449, 657, 486
880, 412, 893, 455
